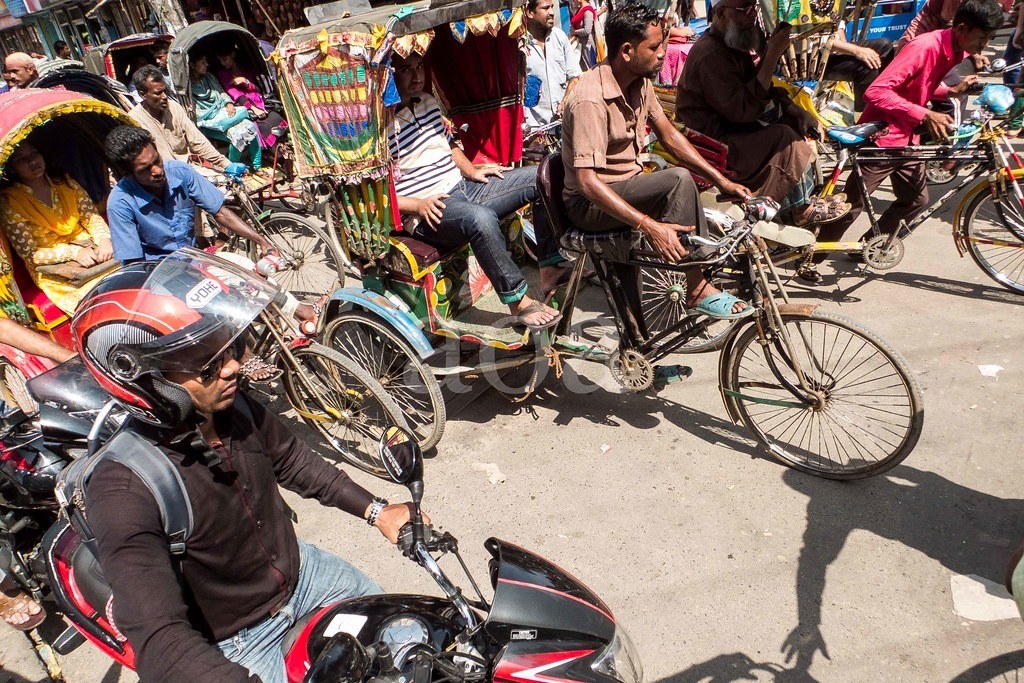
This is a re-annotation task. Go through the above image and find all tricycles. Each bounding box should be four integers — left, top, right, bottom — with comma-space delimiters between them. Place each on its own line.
0, 0, 1024, 484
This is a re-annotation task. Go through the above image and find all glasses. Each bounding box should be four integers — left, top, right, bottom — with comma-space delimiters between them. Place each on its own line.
166, 339, 237, 381
727, 5, 760, 15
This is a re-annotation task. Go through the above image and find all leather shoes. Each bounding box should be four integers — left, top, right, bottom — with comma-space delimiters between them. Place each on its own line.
793, 259, 820, 280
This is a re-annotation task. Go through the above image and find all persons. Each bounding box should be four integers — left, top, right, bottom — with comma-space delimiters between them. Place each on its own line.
71, 247, 431, 683
517, 0, 583, 166
129, 65, 254, 245
0, 136, 125, 320
999, 0, 1024, 138
795, 0, 1005, 282
662, 0, 713, 42
212, 42, 290, 162
188, 46, 262, 172
562, 4, 755, 396
105, 125, 338, 366
895, 1, 991, 175
0, 315, 81, 632
570, 0, 608, 72
379, 51, 598, 327
129, 7, 214, 108
675, 0, 852, 227
786, 21, 895, 125
0, 40, 92, 98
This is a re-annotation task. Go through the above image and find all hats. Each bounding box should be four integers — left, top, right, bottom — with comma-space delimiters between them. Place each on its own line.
711, 1, 720, 8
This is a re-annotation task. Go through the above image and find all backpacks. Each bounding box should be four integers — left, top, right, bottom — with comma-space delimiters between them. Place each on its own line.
54, 414, 191, 575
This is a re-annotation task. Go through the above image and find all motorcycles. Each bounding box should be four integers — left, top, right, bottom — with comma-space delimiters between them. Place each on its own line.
36, 456, 647, 683
0, 355, 131, 601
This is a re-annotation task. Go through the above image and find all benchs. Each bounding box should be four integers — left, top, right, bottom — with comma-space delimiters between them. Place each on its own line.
388, 232, 466, 282
11, 258, 73, 329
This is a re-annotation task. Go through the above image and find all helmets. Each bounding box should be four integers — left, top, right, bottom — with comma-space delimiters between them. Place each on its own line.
74, 258, 236, 429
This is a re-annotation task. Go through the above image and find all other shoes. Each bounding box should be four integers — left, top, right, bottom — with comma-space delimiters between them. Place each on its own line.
254, 170, 268, 178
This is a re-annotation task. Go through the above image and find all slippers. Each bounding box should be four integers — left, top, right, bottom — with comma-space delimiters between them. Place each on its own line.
0, 590, 46, 630
544, 266, 597, 296
796, 200, 851, 227
514, 301, 562, 331
810, 193, 847, 203
686, 287, 755, 320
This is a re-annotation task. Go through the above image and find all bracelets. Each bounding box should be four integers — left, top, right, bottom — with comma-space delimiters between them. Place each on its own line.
636, 214, 648, 229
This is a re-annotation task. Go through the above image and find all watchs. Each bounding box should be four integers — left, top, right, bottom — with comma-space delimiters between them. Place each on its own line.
367, 497, 388, 526
